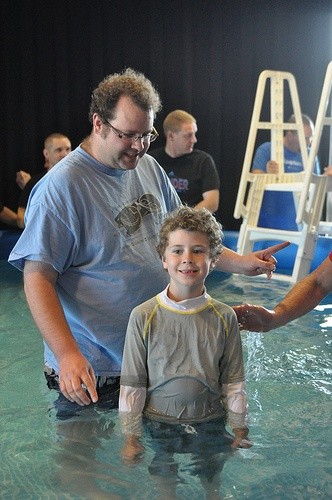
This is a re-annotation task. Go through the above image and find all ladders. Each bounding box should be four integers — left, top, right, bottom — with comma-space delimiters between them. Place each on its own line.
230, 62, 332, 287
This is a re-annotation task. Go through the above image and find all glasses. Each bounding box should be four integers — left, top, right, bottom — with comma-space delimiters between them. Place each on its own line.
96, 113, 159, 143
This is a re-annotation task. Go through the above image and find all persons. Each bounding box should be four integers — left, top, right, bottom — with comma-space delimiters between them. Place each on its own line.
251, 113, 319, 231
323, 167, 332, 177
116, 207, 253, 500
0, 133, 72, 231
146, 110, 220, 217
230, 248, 332, 332
7, 68, 289, 499
